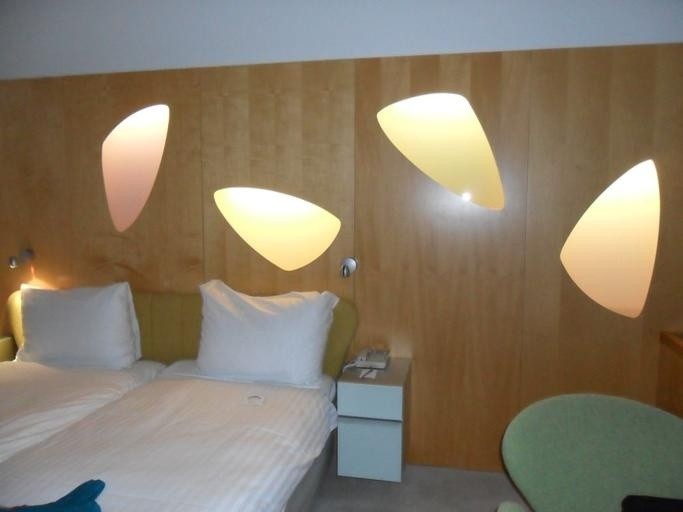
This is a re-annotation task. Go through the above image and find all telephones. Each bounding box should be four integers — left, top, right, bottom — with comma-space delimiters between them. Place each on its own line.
355, 348, 390, 369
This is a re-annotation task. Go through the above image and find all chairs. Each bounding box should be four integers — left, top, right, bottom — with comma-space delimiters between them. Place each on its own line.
495, 390, 681, 510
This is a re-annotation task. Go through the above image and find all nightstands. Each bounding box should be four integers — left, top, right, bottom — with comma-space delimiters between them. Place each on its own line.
333, 356, 415, 484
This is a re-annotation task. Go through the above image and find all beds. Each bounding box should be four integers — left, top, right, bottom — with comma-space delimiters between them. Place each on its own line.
1, 283, 358, 511
